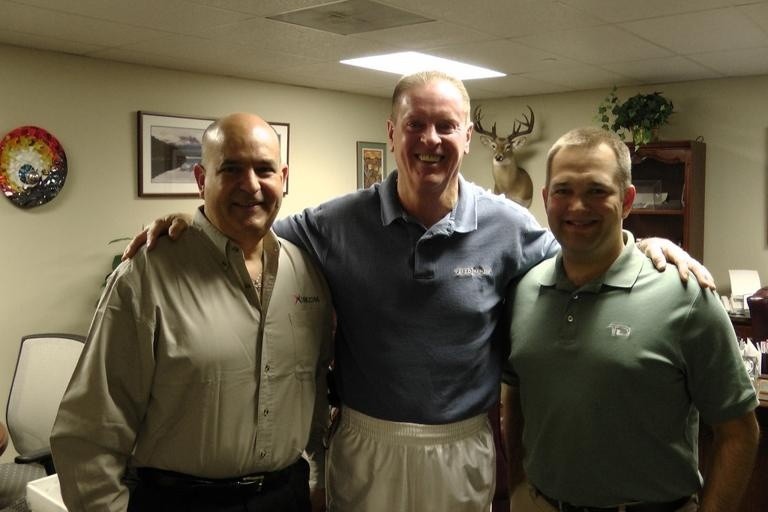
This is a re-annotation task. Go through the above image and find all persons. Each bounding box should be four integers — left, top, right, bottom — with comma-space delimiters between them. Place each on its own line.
50, 113, 335, 512
121, 69, 716, 512
500, 127, 761, 512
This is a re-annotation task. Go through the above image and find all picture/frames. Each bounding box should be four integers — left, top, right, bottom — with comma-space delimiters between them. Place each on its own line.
356, 141, 387, 192
136, 110, 218, 199
264, 121, 290, 195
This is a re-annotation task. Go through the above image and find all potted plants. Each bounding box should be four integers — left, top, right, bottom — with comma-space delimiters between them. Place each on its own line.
597, 83, 675, 152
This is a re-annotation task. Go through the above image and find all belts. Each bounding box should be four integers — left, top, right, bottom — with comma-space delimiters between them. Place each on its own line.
137, 469, 275, 495
535, 488, 692, 512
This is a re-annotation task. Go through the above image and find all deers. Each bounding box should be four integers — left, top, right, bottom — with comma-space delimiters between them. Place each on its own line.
472, 103, 535, 210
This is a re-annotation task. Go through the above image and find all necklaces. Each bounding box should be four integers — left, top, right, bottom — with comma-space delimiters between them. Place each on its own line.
252, 273, 262, 293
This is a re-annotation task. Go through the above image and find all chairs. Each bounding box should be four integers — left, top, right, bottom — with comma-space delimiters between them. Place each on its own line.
0, 332, 89, 509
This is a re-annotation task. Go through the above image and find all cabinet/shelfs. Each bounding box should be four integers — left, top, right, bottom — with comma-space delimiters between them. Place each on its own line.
621, 139, 707, 265
